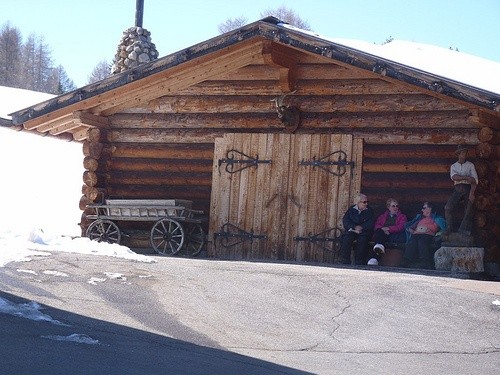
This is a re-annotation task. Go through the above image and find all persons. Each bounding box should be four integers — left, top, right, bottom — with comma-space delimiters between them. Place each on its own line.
367, 199, 408, 266
445, 149, 478, 236
341, 193, 374, 264
404, 201, 446, 269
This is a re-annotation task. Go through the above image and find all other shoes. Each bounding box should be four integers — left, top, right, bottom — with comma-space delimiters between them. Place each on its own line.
368, 258, 379, 265
373, 243, 385, 254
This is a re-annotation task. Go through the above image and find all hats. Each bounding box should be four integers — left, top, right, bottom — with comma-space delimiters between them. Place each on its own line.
454, 144, 469, 153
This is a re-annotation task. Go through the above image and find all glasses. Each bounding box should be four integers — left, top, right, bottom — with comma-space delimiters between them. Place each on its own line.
422, 207, 429, 210
391, 205, 399, 207
360, 200, 369, 204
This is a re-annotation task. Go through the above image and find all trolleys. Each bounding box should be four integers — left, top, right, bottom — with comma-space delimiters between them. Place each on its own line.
84, 199, 210, 258
